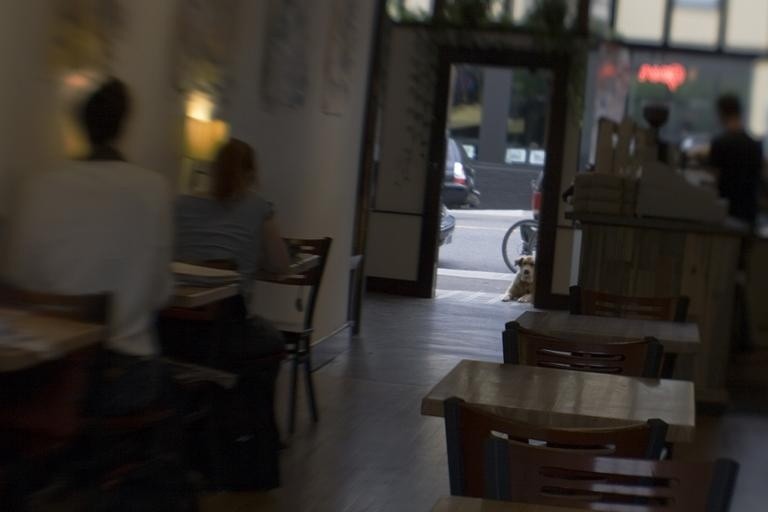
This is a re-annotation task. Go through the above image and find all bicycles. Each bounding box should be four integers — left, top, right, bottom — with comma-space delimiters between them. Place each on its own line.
500, 216, 538, 282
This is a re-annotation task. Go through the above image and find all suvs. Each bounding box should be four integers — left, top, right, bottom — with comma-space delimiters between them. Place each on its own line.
528, 169, 541, 224
442, 139, 483, 210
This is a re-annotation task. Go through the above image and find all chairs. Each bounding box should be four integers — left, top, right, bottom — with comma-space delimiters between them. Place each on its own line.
0, 279, 196, 512
254, 234, 334, 435
420, 284, 740, 510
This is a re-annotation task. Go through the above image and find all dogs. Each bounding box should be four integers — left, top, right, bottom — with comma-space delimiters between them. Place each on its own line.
499, 254, 536, 303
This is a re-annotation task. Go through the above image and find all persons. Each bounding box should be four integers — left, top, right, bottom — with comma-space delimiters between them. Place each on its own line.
0, 76, 282, 494
174, 135, 292, 454
675, 92, 768, 355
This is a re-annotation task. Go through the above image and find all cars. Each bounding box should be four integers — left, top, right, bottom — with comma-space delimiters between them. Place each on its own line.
440, 203, 455, 247
648, 116, 716, 171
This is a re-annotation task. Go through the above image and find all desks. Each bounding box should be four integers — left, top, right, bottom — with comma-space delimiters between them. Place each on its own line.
166, 282, 242, 310
0, 304, 107, 375
